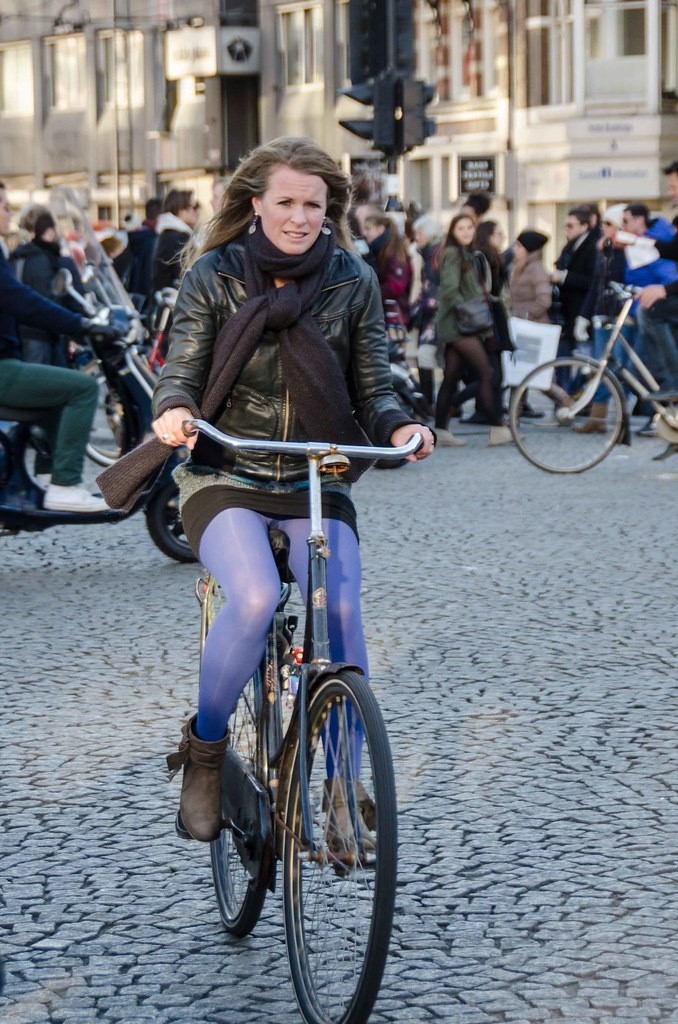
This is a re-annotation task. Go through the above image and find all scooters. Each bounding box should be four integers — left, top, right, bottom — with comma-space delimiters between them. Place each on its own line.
361, 330, 431, 471
1, 182, 200, 566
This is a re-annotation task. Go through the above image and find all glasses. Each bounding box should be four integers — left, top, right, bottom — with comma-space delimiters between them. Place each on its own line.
186, 202, 200, 210
567, 223, 584, 228
623, 218, 640, 224
602, 221, 615, 227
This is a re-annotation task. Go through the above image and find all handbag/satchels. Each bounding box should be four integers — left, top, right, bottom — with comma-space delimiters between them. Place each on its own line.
499, 312, 562, 392
455, 295, 494, 336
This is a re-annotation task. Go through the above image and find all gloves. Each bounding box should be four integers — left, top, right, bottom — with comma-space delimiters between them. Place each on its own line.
79, 307, 128, 343
573, 316, 589, 342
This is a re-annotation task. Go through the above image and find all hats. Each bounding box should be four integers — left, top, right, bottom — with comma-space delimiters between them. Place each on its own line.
602, 203, 630, 226
518, 232, 547, 252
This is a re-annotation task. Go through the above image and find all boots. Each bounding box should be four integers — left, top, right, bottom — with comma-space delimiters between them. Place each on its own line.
571, 402, 608, 434
166, 716, 232, 843
322, 779, 376, 877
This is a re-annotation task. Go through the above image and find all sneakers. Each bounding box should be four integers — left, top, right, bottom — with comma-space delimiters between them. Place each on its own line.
43, 483, 112, 512
36, 473, 92, 495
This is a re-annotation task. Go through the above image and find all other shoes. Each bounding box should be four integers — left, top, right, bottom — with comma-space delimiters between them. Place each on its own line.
636, 422, 660, 436
520, 404, 544, 418
653, 443, 678, 461
435, 429, 468, 447
648, 388, 678, 401
459, 410, 503, 426
490, 426, 526, 445
432, 400, 461, 417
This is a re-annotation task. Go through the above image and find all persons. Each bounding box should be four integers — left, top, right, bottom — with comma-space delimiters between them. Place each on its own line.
0, 181, 120, 511
95, 137, 438, 878
126, 177, 228, 359
8, 203, 85, 368
355, 204, 678, 445
635, 165, 678, 461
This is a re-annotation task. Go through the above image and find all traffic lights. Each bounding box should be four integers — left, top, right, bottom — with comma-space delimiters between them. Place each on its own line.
336, 74, 399, 150
401, 78, 438, 152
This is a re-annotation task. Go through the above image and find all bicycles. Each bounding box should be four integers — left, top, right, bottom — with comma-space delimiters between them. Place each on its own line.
507, 279, 678, 476
174, 418, 425, 1024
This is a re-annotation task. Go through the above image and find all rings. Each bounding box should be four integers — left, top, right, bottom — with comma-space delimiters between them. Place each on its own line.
162, 434, 169, 440
